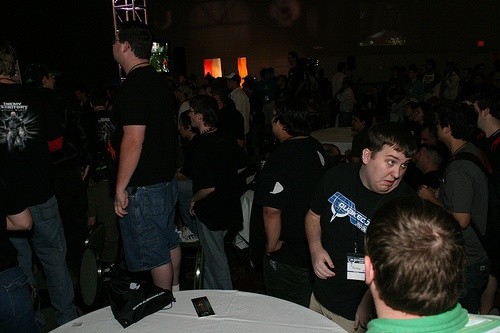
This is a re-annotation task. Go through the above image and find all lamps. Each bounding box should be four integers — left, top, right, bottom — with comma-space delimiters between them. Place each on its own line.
237, 57, 248, 88
203, 58, 221, 80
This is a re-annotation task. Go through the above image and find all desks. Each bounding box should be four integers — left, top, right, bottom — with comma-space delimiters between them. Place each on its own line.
309, 128, 354, 155
48, 290, 348, 333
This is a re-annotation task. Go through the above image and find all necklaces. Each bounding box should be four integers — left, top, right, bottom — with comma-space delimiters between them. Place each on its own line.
128, 61, 150, 73
200, 126, 216, 134
0, 76, 16, 82
279, 134, 298, 142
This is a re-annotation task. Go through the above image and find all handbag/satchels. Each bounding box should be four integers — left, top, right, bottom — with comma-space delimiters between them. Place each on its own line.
225, 231, 262, 274
105, 271, 175, 329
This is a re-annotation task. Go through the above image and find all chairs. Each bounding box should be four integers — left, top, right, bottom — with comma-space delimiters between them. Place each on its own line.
176, 216, 204, 290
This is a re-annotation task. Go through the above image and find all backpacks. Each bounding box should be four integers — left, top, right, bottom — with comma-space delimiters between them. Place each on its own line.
447, 146, 500, 270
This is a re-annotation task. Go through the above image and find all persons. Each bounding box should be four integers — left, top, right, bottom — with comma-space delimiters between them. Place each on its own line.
417, 102, 489, 315
88, 163, 127, 270
28, 1, 499, 181
175, 109, 202, 243
0, 39, 80, 328
0, 169, 45, 332
112, 20, 183, 294
254, 93, 327, 307
182, 95, 244, 290
23, 62, 90, 302
364, 196, 500, 333
304, 121, 423, 333
469, 89, 500, 316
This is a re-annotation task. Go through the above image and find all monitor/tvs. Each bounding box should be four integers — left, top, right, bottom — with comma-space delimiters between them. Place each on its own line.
149, 39, 170, 74
